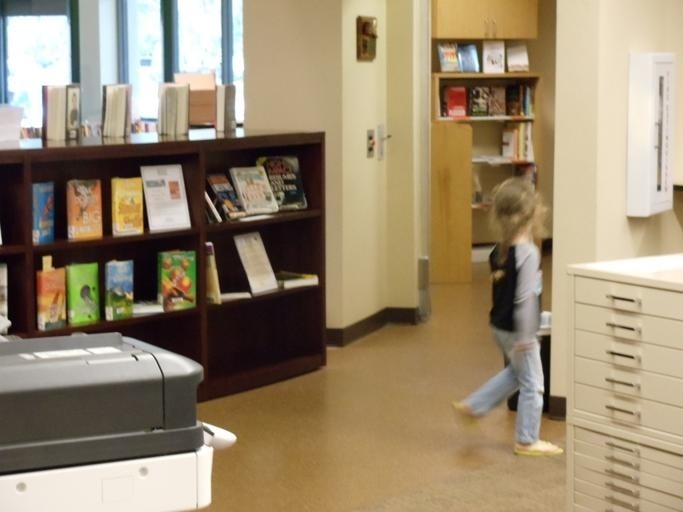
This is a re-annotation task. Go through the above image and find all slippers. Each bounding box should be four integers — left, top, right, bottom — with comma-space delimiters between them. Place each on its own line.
452, 402, 482, 437
513, 442, 564, 457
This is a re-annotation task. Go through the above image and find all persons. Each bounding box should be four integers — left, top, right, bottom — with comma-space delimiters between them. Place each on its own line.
450, 176, 566, 457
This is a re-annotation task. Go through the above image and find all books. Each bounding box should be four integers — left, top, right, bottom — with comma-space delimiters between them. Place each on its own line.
37, 250, 197, 330
206, 156, 309, 222
205, 232, 319, 305
155, 82, 191, 136
32, 164, 192, 245
437, 42, 535, 164
40, 84, 80, 143
99, 84, 135, 141
0, 263, 8, 334
212, 84, 238, 133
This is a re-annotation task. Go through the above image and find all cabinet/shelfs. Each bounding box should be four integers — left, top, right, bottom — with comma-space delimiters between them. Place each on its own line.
0, 130, 329, 403
566, 252, 683, 512
430, 0, 543, 285
